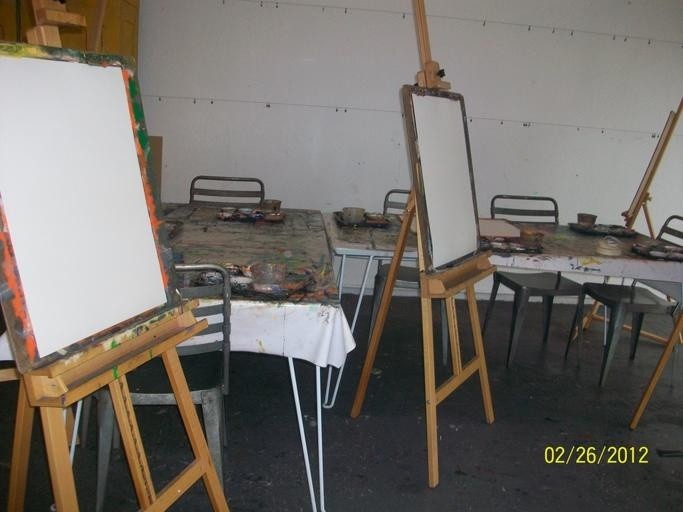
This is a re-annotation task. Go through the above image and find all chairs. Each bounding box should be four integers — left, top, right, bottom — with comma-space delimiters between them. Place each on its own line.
188, 176, 266, 210
483, 195, 585, 370
566, 212, 683, 389
369, 190, 450, 369
92, 263, 231, 512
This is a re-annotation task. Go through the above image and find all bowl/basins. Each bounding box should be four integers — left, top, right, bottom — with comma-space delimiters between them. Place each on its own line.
250, 262, 288, 295
259, 199, 281, 213
520, 230, 545, 245
220, 264, 239, 273
221, 206, 236, 214
342, 207, 366, 225
199, 271, 221, 284
577, 211, 598, 230
229, 275, 251, 289
257, 210, 288, 221
239, 264, 251, 276
239, 208, 252, 214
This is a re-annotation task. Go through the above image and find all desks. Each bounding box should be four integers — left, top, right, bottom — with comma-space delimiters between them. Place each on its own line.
322, 211, 683, 408
0, 201, 360, 512
